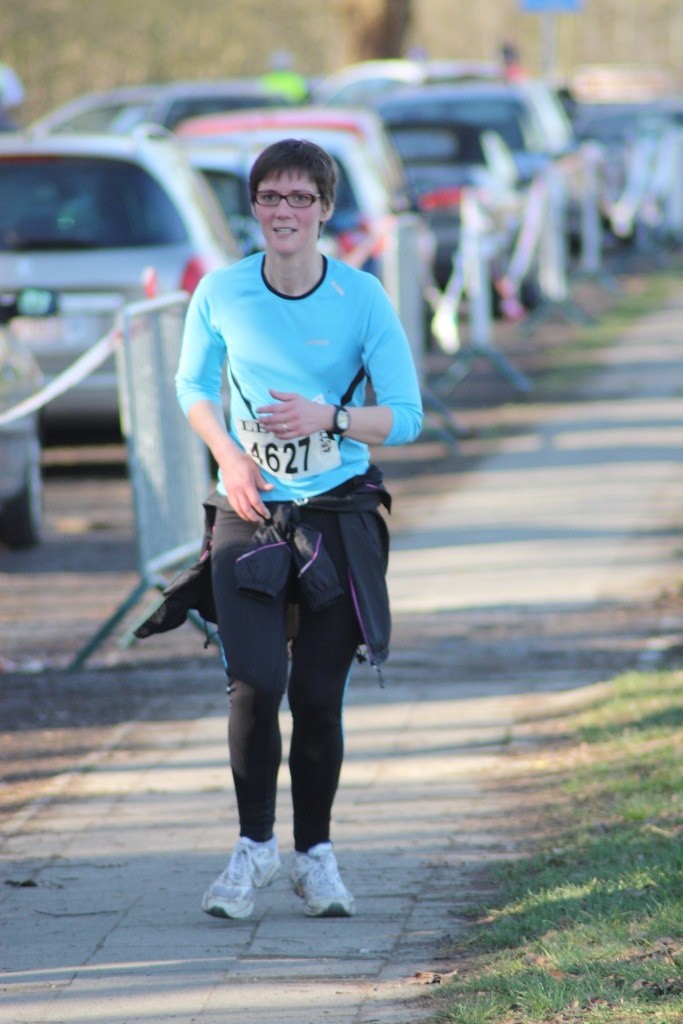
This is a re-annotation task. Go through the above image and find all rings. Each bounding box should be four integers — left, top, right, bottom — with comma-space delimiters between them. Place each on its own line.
283, 423, 288, 433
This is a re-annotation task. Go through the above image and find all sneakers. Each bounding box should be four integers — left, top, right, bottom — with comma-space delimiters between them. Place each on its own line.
202, 832, 281, 919
291, 840, 356, 918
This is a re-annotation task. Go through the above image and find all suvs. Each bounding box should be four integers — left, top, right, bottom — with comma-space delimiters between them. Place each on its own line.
0, 121, 251, 444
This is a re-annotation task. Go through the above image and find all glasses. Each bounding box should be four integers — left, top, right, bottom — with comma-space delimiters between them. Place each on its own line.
252, 189, 321, 208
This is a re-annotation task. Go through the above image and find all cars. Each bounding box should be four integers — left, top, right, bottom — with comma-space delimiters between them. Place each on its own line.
0, 287, 62, 546
22, 59, 683, 380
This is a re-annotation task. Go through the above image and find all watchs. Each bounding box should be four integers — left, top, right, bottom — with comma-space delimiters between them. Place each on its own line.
330, 404, 350, 435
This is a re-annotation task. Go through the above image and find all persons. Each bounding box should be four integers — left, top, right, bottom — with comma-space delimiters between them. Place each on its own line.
172, 140, 423, 918
493, 44, 530, 81
262, 51, 310, 105
